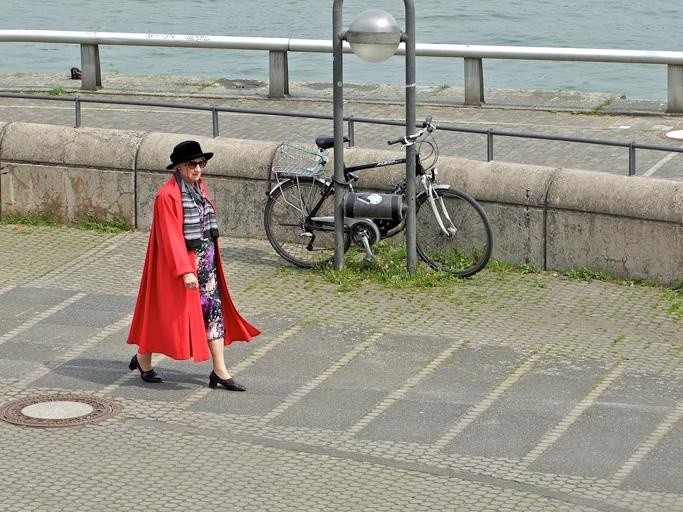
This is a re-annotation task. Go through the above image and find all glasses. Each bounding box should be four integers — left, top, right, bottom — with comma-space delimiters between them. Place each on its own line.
185, 160, 208, 169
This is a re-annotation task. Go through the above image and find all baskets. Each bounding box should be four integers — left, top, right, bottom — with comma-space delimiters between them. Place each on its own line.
272, 134, 329, 176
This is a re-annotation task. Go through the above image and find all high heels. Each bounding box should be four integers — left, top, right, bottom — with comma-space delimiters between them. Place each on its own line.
128, 355, 162, 382
209, 371, 245, 391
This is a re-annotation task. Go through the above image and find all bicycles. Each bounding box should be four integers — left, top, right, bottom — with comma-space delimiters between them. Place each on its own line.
264, 115, 492, 279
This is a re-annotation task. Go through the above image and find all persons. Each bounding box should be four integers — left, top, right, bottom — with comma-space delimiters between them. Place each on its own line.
126, 141, 246, 393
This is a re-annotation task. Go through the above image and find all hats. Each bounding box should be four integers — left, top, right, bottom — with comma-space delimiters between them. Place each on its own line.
167, 141, 214, 169
